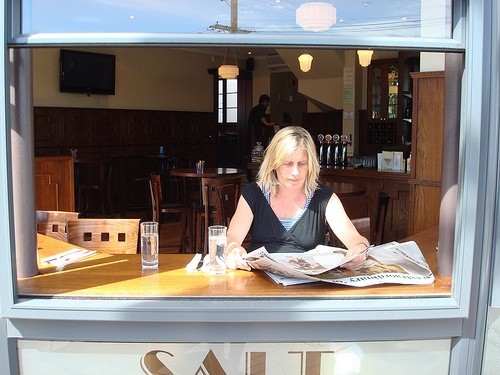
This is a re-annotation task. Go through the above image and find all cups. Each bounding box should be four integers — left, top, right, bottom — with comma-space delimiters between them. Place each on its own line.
208, 226, 227, 275
349, 154, 377, 169
140, 221, 159, 269
196, 161, 205, 174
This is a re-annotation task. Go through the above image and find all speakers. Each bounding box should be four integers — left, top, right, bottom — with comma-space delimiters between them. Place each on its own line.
246, 58, 254, 71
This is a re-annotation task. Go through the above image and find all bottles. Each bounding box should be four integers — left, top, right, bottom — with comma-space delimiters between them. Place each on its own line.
251, 141, 265, 163
316, 135, 348, 165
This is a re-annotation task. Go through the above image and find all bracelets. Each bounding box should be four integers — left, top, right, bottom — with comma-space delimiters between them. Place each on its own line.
227, 242, 239, 251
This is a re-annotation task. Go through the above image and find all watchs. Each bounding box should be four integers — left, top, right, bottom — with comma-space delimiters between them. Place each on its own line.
357, 243, 369, 259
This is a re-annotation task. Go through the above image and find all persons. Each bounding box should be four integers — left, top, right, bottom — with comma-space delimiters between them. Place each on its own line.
225, 126, 370, 271
248, 94, 274, 146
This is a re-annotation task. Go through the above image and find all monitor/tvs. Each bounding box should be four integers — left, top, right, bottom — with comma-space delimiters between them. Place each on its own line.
60, 49, 116, 96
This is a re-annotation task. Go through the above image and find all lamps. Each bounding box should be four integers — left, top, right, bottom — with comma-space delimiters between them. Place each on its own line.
357, 50, 373, 67
298, 48, 314, 73
218, 47, 239, 78
296, 2, 337, 32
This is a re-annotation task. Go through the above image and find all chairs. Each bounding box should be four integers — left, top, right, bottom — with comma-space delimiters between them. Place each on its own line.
36, 159, 391, 256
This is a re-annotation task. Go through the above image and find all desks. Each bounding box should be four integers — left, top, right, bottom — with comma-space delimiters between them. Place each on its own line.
16, 224, 451, 295
170, 168, 243, 254
219, 182, 366, 246
